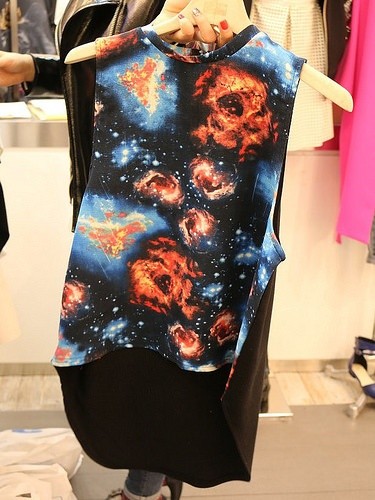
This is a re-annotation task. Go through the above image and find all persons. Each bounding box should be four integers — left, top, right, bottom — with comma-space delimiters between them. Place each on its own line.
0, 47, 185, 500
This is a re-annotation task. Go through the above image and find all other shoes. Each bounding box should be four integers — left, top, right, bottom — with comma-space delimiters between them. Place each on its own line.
348, 349, 375, 400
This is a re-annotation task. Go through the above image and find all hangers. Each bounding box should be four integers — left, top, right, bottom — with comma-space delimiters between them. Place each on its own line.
60, 0, 357, 114
